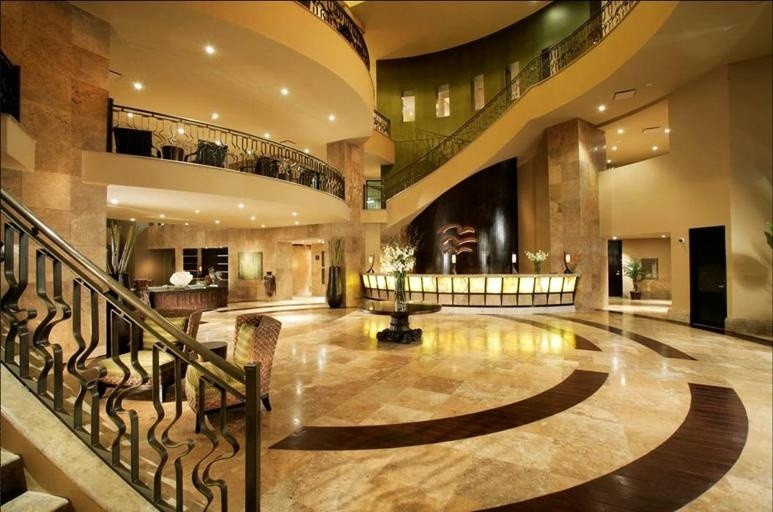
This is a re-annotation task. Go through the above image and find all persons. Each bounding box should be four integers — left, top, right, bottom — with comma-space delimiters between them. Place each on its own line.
190, 270, 213, 286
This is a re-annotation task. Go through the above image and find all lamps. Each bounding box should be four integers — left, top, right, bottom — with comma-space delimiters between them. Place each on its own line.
562, 250, 573, 273
450, 248, 457, 274
511, 249, 517, 273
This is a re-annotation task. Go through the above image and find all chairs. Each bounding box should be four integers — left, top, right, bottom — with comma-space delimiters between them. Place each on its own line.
87, 307, 282, 433
112, 125, 320, 191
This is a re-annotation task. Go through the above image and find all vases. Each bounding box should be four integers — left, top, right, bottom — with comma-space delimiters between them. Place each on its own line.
393, 271, 409, 312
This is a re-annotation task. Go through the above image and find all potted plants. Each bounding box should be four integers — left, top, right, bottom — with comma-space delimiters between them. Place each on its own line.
324, 238, 344, 307
621, 256, 646, 299
106, 219, 144, 357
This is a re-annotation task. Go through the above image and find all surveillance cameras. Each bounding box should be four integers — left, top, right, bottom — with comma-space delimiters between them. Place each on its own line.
678, 238, 685, 244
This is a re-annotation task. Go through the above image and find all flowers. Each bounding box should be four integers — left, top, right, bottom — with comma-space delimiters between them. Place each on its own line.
377, 241, 416, 312
524, 248, 550, 273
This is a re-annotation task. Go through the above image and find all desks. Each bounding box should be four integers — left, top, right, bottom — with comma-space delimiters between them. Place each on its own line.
359, 300, 442, 344
